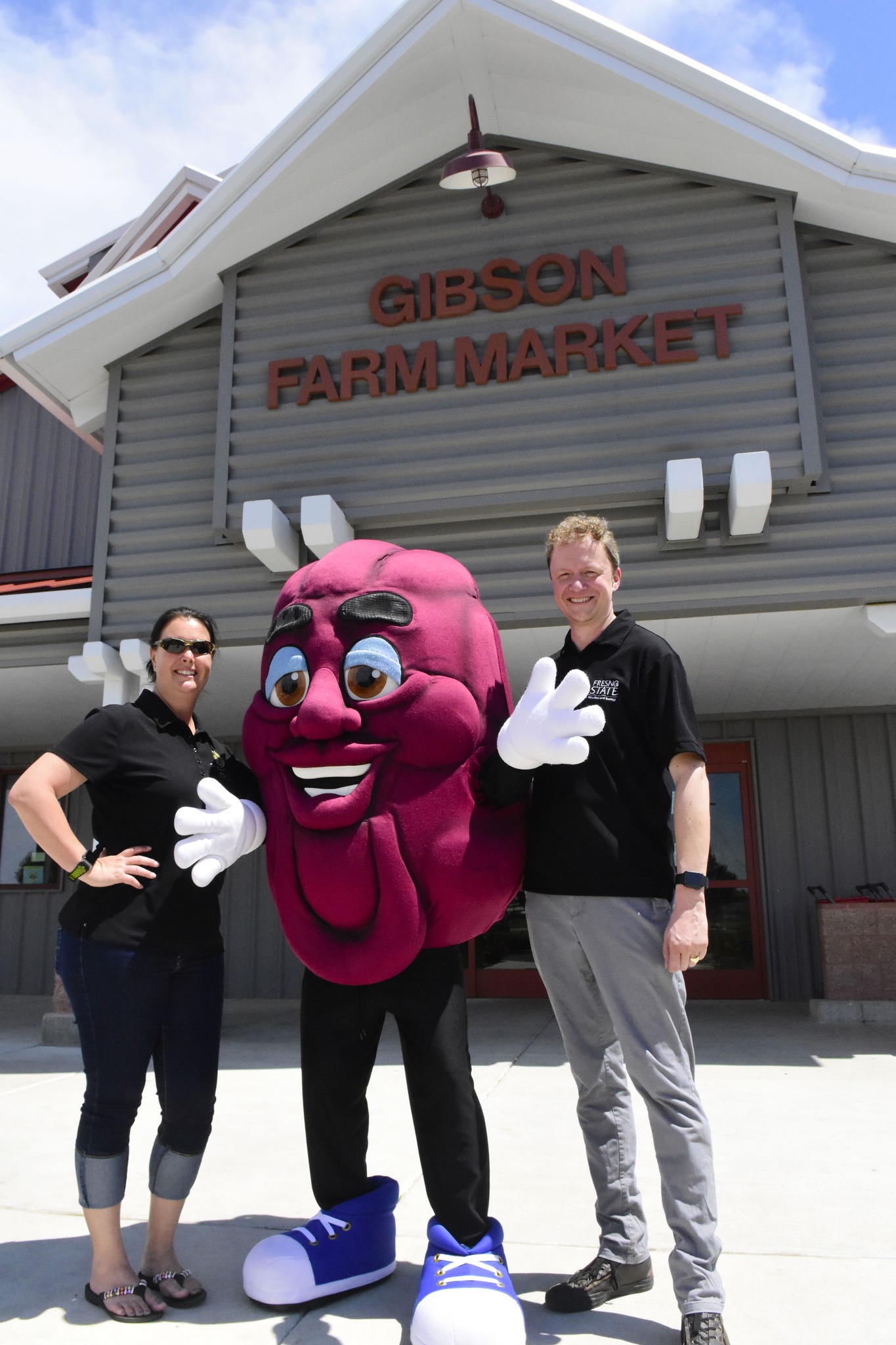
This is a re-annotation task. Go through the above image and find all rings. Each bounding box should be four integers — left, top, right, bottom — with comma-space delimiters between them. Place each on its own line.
690, 957, 700, 964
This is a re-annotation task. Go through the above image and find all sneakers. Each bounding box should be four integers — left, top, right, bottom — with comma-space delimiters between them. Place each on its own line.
544, 1255, 656, 1314
680, 1312, 731, 1345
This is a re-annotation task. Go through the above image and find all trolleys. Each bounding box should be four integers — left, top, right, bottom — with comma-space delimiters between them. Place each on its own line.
807, 881, 896, 904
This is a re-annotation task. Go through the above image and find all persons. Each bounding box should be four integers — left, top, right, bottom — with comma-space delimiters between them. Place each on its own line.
240, 538, 524, 1345
6, 608, 269, 1324
477, 512, 728, 1345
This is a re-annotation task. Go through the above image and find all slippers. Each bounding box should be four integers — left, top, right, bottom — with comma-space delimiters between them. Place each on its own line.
137, 1270, 207, 1309
84, 1278, 166, 1323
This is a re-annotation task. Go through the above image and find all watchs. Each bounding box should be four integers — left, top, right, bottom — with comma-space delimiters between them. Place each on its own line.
674, 871, 709, 890
66, 849, 97, 882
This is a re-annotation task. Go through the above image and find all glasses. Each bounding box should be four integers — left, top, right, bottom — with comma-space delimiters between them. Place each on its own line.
152, 637, 216, 656
